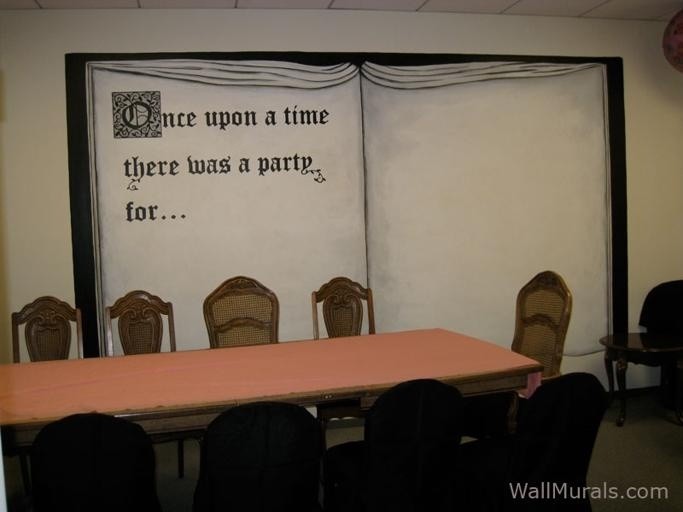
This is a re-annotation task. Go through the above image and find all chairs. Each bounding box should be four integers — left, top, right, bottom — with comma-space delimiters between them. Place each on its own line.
0, 260, 682, 510
